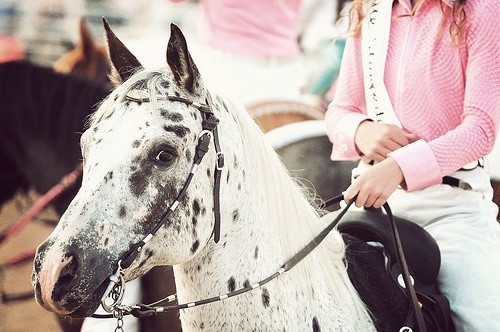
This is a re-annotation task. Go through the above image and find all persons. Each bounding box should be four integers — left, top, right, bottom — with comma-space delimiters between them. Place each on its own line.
324, 0, 500, 332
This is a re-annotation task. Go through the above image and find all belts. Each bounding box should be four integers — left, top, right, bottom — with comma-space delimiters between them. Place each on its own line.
368, 159, 473, 191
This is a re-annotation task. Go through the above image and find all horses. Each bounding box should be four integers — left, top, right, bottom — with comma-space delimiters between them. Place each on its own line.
0, 15, 452, 332
